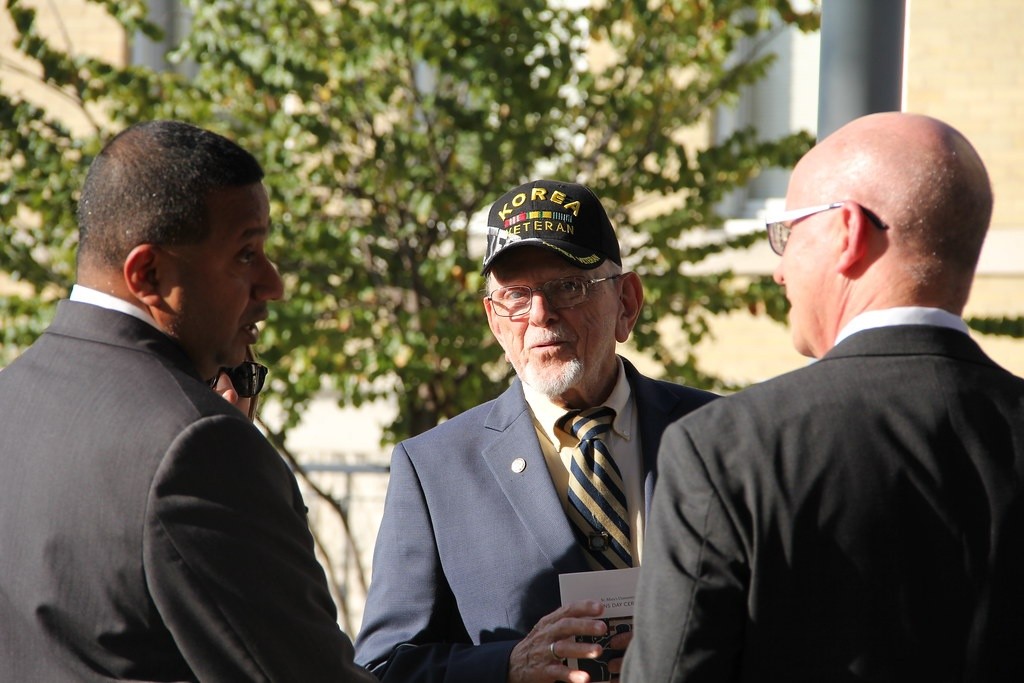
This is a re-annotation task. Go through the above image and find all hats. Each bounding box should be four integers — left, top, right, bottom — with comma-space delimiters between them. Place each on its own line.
480, 179, 624, 277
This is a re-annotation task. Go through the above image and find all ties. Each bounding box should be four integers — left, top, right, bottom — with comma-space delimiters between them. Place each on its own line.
556, 406, 634, 683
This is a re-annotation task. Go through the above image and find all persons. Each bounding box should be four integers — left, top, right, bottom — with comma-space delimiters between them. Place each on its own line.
0, 121, 355, 683
355, 179, 725, 683
619, 112, 1024, 683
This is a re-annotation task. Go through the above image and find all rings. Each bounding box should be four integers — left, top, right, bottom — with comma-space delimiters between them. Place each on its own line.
551, 643, 564, 661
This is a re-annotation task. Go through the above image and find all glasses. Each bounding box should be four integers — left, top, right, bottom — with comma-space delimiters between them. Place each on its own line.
488, 272, 624, 317
206, 360, 268, 399
764, 201, 889, 256
